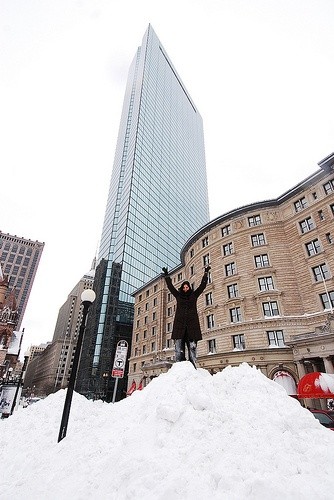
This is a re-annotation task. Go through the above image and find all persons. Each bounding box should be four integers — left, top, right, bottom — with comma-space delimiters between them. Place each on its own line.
161, 265, 212, 371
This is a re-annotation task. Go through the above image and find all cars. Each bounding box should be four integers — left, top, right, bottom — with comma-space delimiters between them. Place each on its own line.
311, 410, 334, 432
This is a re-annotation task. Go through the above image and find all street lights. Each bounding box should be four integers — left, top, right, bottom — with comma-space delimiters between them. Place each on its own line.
10, 349, 32, 415
57, 289, 97, 442
7, 367, 13, 385
